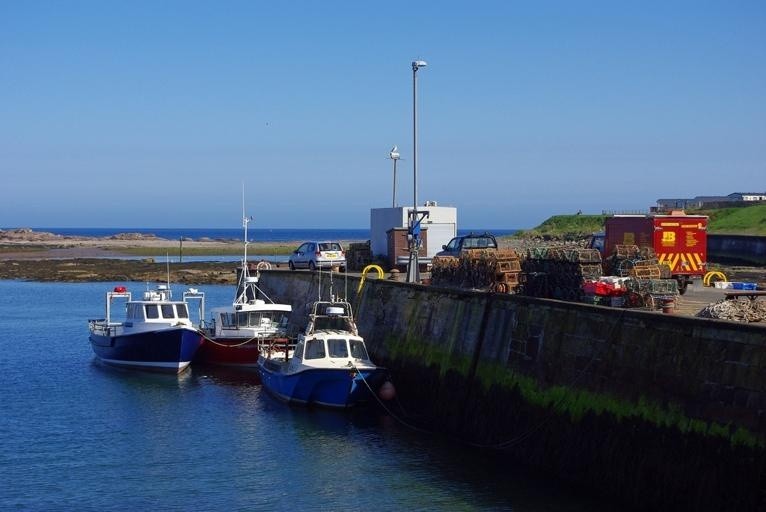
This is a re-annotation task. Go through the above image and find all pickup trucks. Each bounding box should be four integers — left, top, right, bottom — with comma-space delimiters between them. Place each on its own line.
436, 232, 499, 257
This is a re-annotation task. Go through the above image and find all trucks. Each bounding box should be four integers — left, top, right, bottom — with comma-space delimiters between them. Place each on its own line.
590, 214, 710, 295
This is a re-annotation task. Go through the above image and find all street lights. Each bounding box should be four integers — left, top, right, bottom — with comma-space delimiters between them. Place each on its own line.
406, 60, 426, 284
